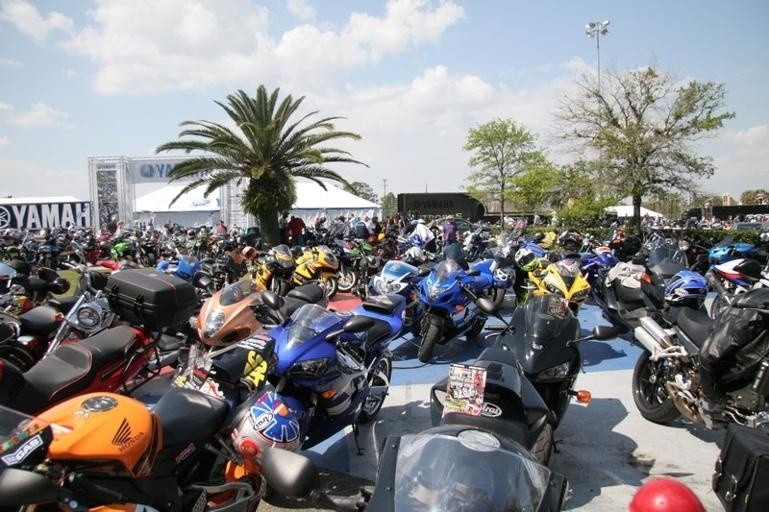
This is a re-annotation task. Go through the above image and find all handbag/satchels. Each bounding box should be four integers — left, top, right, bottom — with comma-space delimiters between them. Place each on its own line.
709, 424, 769, 511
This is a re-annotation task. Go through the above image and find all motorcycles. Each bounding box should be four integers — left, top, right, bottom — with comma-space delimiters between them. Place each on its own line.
1, 226, 768, 512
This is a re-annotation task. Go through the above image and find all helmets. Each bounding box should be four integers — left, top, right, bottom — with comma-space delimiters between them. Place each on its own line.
41, 222, 197, 245
514, 246, 539, 271
378, 232, 385, 243
662, 269, 709, 308
367, 235, 377, 244
562, 228, 583, 250
242, 246, 257, 260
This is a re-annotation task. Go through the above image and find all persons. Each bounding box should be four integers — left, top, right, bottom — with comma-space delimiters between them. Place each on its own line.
279, 211, 528, 253
1, 216, 244, 268
603, 211, 769, 268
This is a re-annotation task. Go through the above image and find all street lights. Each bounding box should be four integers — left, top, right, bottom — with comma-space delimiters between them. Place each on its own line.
583, 18, 611, 199
